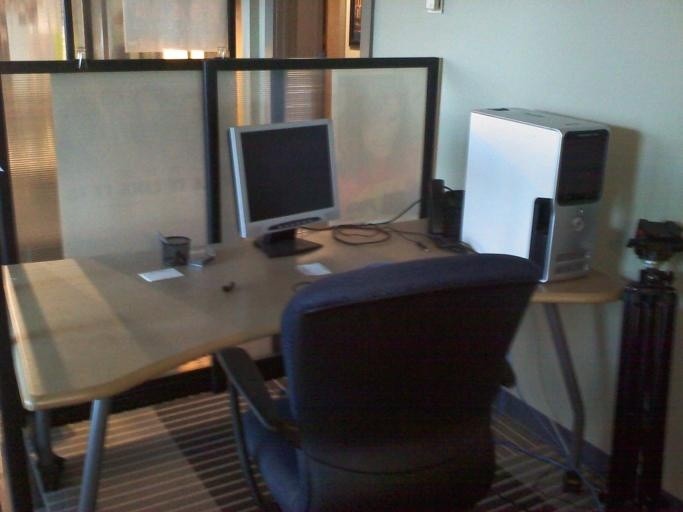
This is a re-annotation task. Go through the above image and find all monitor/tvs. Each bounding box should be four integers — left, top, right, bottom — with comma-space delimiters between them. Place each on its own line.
226, 117, 340, 259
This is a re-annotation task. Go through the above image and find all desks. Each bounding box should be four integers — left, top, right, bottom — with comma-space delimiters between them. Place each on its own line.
1, 227, 634, 512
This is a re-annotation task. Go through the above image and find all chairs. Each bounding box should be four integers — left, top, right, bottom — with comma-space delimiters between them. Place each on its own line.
213, 253, 542, 512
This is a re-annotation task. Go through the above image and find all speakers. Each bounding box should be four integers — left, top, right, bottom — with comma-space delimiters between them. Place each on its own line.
426, 178, 461, 249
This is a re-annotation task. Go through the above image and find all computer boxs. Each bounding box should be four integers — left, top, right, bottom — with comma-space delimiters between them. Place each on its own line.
459, 107, 610, 285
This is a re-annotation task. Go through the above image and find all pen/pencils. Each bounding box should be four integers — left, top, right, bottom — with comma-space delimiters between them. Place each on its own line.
154, 230, 187, 265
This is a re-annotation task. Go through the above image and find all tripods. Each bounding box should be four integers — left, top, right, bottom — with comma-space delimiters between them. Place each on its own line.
609, 218, 682, 510
